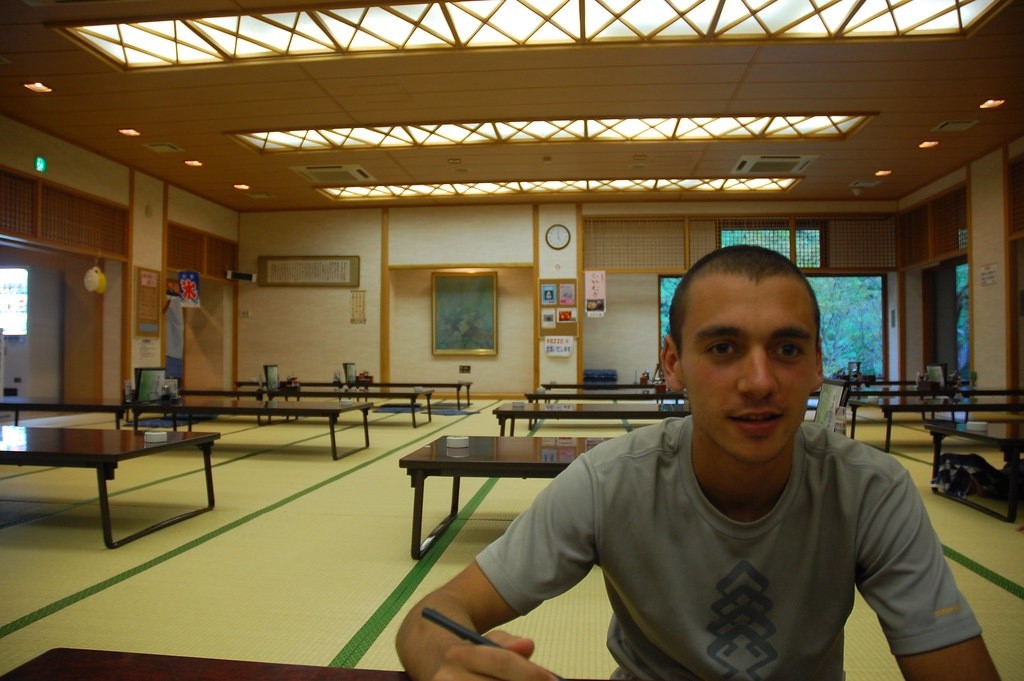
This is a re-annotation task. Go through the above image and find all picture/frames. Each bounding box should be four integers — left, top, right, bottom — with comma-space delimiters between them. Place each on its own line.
257, 255, 360, 287
431, 271, 497, 356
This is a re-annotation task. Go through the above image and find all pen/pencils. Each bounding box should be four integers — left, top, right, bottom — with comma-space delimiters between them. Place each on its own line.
420, 606, 565, 681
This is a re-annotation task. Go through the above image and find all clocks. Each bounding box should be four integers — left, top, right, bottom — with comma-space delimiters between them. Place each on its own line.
545, 224, 570, 249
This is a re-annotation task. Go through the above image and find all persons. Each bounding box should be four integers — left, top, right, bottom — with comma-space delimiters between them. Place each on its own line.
395, 244, 1004, 681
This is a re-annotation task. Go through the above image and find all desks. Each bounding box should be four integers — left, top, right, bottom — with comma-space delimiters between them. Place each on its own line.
400, 379, 1024, 560
0, 426, 220, 549
0, 647, 614, 681
0, 381, 473, 460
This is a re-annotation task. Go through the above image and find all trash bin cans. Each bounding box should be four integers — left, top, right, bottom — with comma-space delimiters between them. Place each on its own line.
582, 369, 618, 385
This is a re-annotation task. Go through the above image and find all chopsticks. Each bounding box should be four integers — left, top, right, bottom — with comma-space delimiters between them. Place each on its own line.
420, 608, 570, 681
